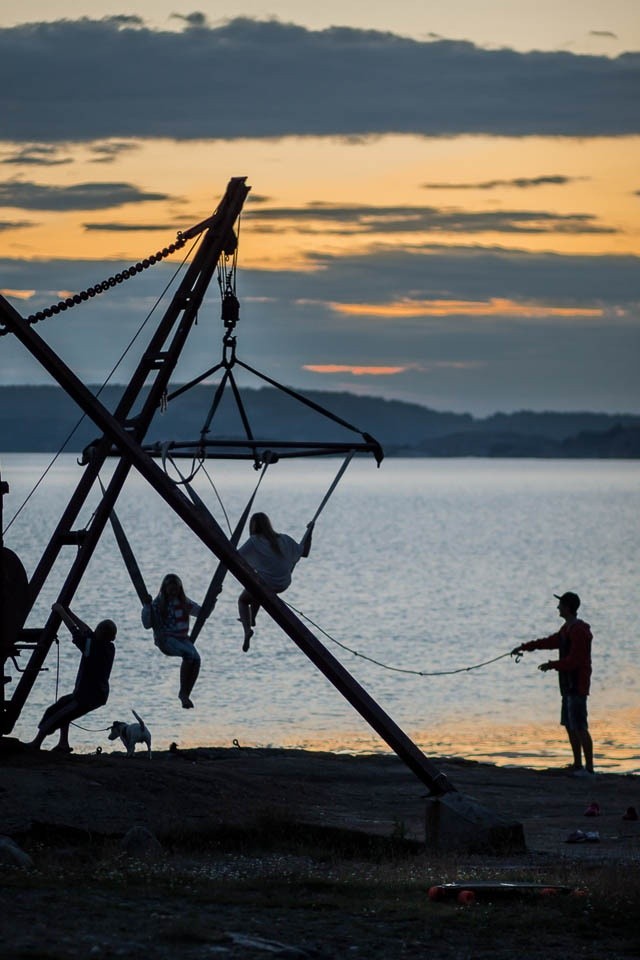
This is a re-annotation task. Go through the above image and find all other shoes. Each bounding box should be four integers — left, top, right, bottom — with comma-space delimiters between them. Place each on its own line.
51, 746, 73, 754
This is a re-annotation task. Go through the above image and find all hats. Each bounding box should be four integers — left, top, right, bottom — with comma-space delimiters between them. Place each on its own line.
553, 592, 581, 606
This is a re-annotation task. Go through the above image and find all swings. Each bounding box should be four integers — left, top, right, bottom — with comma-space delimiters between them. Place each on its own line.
162, 447, 357, 595
82, 450, 274, 655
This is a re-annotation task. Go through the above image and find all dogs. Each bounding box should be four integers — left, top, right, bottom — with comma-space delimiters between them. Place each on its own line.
108, 709, 152, 760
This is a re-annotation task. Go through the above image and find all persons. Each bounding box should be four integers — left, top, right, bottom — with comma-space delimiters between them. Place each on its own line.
26, 602, 118, 753
509, 591, 594, 773
141, 574, 223, 709
235, 512, 315, 652
